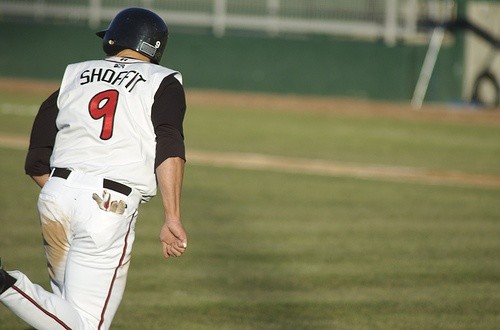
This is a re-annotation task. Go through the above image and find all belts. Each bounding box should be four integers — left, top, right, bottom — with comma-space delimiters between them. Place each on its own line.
48, 168, 132, 197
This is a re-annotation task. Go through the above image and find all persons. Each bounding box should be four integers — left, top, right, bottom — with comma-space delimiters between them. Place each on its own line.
0, 7, 188, 329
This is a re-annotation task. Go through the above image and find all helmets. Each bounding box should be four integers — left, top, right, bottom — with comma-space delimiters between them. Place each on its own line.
95, 7, 168, 65
